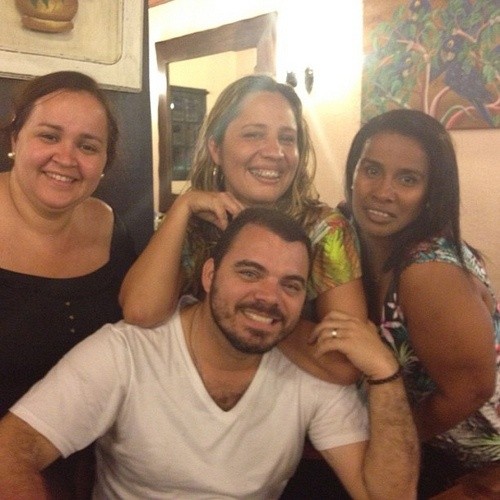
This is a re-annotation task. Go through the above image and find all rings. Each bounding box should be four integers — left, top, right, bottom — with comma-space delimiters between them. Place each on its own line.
332, 329, 337, 338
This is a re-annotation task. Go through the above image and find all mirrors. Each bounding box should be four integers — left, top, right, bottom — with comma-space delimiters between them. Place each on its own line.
156, 9, 278, 215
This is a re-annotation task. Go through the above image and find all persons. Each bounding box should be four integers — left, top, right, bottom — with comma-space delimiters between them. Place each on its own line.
0, 208, 421, 499
0, 72, 140, 420
119, 76, 368, 388
336, 108, 500, 479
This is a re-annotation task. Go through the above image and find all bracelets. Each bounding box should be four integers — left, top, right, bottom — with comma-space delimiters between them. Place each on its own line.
366, 365, 405, 384
